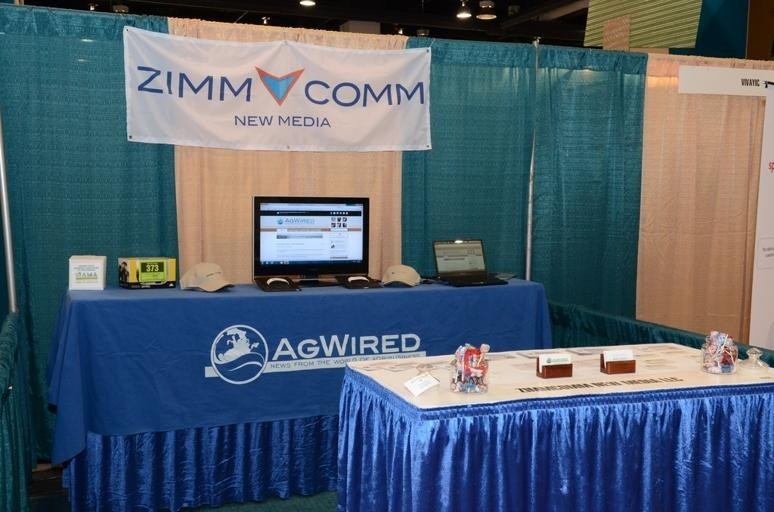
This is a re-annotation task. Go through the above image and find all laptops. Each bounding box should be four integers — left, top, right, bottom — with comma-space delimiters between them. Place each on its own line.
432, 239, 509, 287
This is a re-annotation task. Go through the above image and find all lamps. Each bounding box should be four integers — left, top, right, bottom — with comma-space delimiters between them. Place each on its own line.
455, 0, 497, 20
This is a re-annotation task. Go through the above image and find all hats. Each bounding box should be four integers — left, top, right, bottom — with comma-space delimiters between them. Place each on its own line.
382, 264, 421, 287
180, 262, 235, 292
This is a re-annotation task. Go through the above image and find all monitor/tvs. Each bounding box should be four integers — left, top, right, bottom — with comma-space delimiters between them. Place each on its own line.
253, 195, 369, 287
